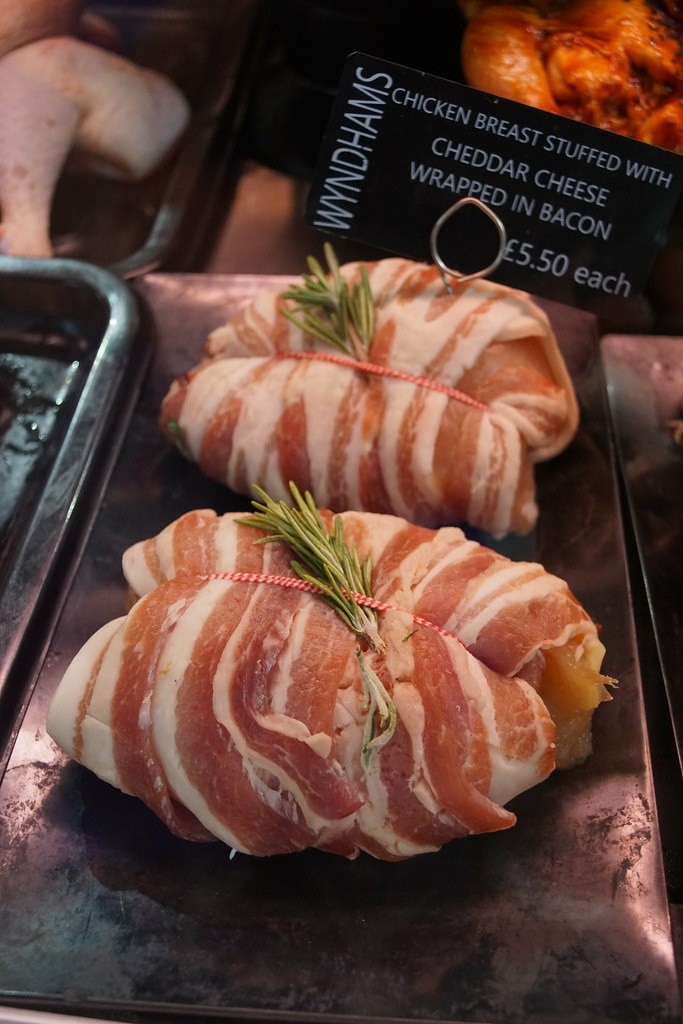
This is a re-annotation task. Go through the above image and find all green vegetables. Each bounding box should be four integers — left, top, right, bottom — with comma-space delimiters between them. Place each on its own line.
231, 478, 400, 772
277, 240, 377, 387
